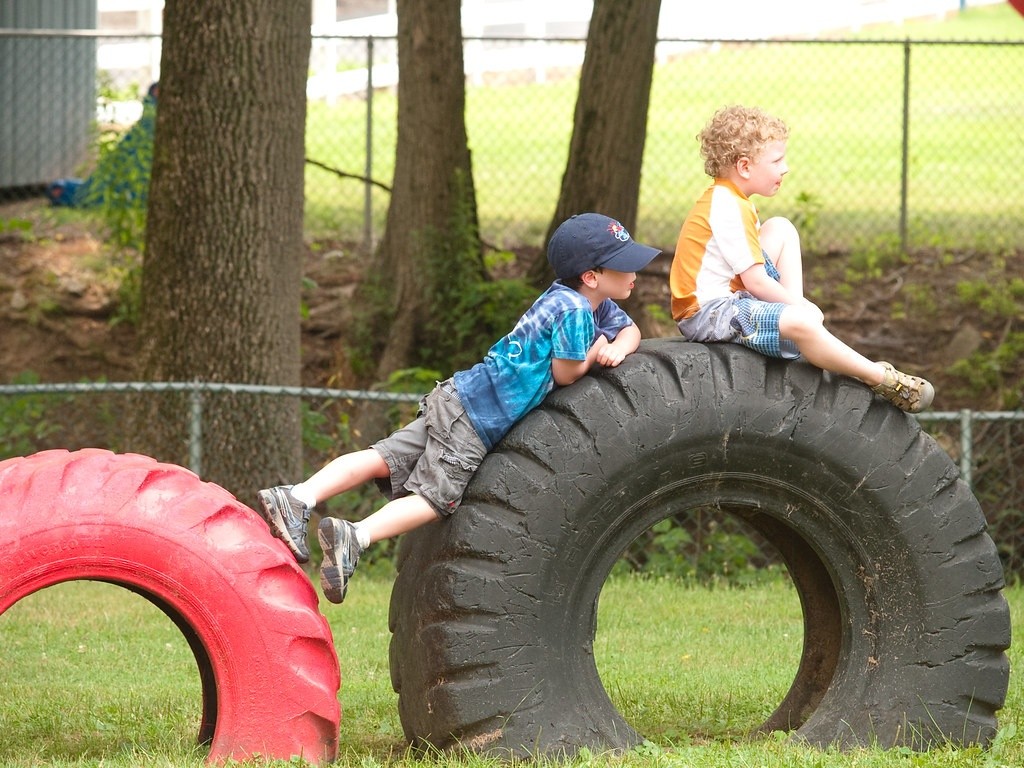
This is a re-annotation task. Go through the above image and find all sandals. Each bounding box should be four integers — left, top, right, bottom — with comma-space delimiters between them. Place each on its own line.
869, 359, 935, 413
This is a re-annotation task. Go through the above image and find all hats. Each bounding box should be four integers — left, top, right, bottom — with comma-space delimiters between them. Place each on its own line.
547, 212, 662, 280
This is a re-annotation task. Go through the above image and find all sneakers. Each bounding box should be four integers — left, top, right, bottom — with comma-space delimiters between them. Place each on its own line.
256, 484, 311, 564
317, 517, 363, 604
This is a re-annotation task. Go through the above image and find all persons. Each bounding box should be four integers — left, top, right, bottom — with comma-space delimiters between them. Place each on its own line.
668, 103, 935, 416
257, 212, 662, 610
